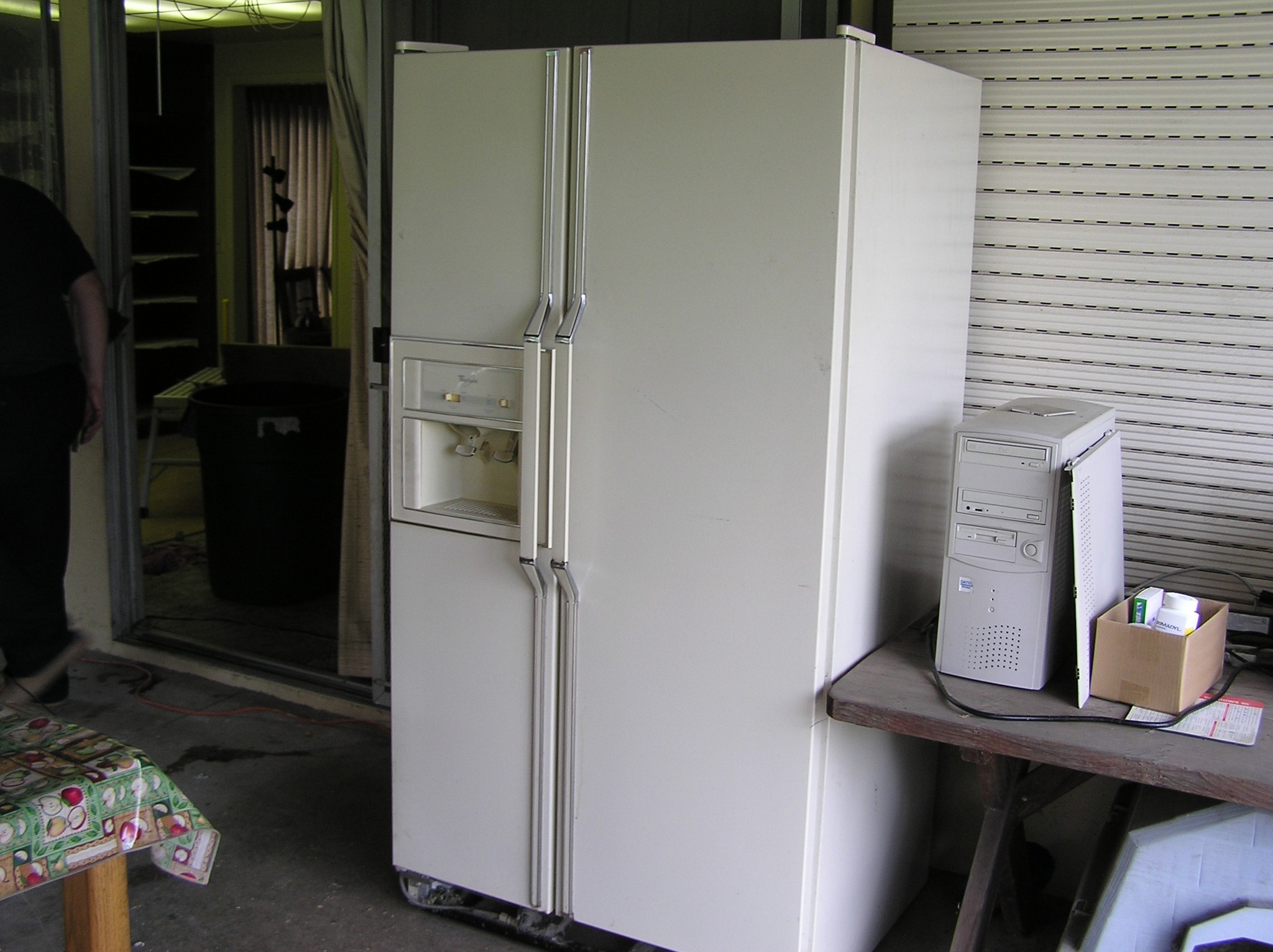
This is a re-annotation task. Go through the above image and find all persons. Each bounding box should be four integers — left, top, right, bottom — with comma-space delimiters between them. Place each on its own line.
1, 170, 108, 706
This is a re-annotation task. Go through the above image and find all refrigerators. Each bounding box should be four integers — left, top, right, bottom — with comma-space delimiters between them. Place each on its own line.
373, 27, 979, 951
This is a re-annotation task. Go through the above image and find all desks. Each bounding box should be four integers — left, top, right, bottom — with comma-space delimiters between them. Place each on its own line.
0, 711, 218, 951
826, 597, 1273, 952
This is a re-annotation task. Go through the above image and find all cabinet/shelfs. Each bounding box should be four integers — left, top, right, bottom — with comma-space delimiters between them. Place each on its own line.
131, 163, 202, 351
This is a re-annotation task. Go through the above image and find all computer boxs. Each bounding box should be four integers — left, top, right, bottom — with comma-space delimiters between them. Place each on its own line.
932, 396, 1115, 691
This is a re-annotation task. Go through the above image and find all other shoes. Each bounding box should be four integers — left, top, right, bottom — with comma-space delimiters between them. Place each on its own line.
30, 687, 69, 708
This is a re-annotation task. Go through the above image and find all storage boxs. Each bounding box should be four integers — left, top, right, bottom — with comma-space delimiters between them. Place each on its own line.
1089, 587, 1231, 718
